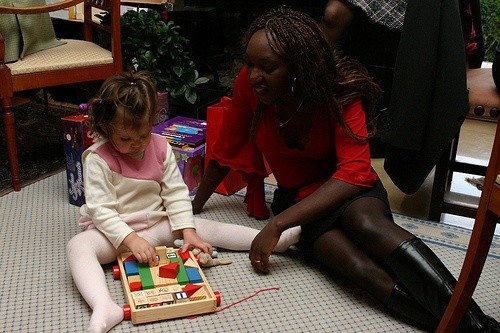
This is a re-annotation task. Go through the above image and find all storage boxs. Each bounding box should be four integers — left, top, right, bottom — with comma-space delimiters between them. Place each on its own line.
60, 115, 95, 207
151, 117, 207, 195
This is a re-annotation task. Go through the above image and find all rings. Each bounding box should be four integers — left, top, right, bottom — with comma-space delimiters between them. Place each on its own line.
255, 260, 261, 263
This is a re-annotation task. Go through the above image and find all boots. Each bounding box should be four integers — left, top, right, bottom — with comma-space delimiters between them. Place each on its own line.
387, 236, 500, 333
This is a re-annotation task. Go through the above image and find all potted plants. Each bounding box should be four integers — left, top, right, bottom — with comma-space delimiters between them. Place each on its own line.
121, 9, 209, 126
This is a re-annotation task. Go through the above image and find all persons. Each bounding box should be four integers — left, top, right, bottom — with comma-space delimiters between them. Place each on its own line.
66, 70, 301, 333
192, 0, 500, 333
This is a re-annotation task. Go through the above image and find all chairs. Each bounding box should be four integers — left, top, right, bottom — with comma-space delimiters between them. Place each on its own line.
421, 86, 500, 333
0, 0, 122, 193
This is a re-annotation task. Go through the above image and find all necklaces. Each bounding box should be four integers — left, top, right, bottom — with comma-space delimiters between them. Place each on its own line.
280, 97, 306, 127
